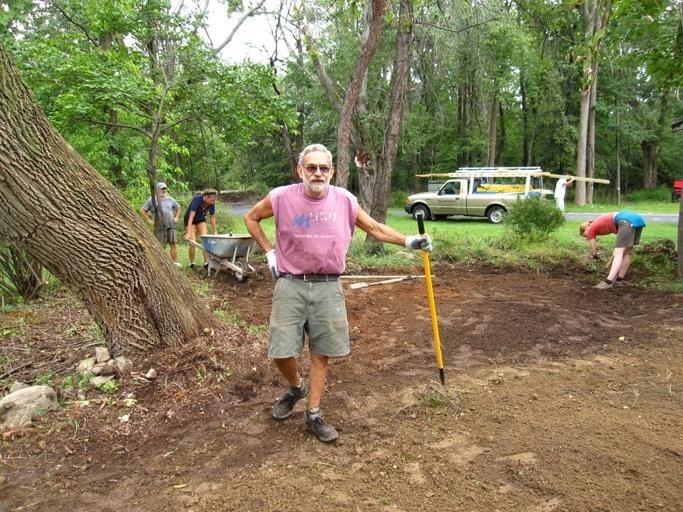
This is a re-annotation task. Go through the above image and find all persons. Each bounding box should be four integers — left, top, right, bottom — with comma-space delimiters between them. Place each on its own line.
182, 188, 218, 269
578, 211, 645, 291
242, 144, 432, 442
140, 182, 181, 266
553, 174, 574, 211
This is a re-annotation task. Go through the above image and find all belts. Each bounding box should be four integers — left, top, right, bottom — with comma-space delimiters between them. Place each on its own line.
280, 272, 339, 282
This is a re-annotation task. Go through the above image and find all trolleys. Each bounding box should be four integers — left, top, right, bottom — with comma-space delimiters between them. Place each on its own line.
183, 232, 264, 283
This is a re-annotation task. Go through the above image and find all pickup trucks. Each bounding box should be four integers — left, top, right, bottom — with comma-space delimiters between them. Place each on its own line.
403, 177, 530, 223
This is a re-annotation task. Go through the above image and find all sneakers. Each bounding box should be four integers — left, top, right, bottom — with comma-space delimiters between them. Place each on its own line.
305, 408, 338, 442
272, 379, 308, 419
595, 280, 614, 290
615, 280, 624, 286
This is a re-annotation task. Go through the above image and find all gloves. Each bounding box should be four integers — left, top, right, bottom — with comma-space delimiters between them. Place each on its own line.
265, 249, 281, 279
405, 233, 432, 251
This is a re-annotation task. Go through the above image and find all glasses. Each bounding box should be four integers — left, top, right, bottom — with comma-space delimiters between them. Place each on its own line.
303, 163, 330, 173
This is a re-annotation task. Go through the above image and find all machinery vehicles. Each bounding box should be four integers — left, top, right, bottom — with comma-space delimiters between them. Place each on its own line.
671, 179, 682, 203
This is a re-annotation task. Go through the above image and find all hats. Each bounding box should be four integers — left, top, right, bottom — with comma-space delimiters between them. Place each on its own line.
157, 183, 167, 189
